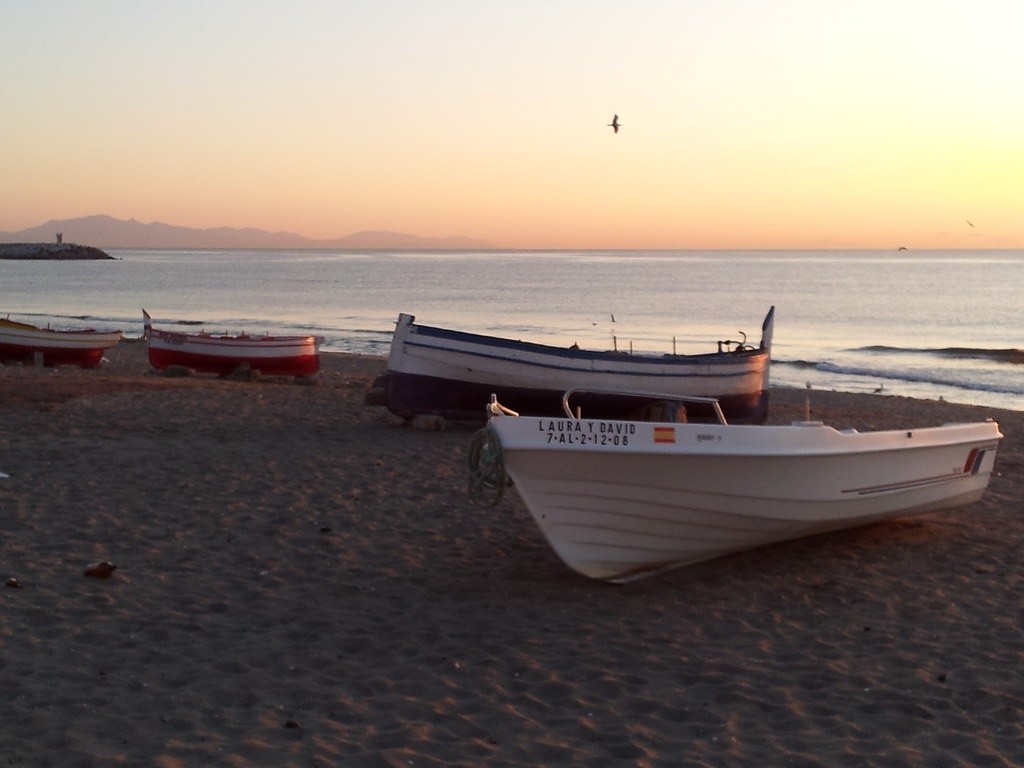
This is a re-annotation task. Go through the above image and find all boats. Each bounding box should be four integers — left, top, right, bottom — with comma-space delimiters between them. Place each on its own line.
381, 313, 769, 419
148, 327, 320, 376
0, 318, 122, 366
485, 387, 1005, 584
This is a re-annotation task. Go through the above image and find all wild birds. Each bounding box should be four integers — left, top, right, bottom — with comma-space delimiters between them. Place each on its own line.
872, 383, 884, 395
966, 219, 974, 228
898, 247, 908, 252
606, 114, 622, 134
